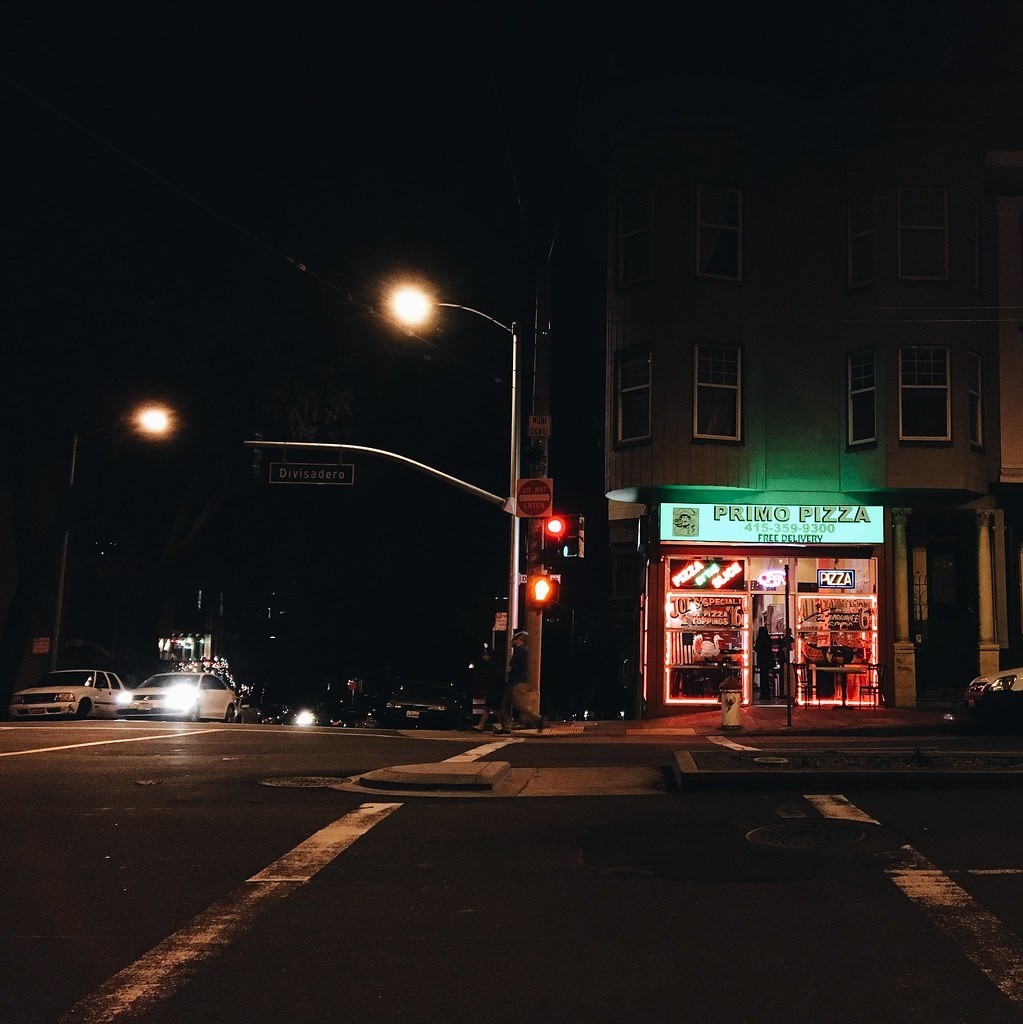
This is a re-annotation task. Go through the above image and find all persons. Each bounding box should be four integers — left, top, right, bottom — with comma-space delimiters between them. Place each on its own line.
753, 626, 775, 702
473, 648, 504, 731
493, 632, 547, 737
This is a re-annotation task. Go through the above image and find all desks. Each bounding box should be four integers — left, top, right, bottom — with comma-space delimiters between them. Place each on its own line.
809, 666, 866, 711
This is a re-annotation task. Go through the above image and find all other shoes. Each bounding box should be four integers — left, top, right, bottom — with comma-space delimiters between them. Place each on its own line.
759, 697, 771, 700
537, 717, 545, 731
494, 729, 511, 737
472, 726, 485, 731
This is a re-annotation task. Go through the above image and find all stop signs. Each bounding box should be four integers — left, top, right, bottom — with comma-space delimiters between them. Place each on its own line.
517, 479, 553, 518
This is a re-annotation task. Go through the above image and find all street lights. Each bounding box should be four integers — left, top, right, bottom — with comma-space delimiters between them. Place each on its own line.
384, 286, 522, 684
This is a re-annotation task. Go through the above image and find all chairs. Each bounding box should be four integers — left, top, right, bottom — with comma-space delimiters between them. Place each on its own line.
792, 662, 821, 712
859, 662, 889, 713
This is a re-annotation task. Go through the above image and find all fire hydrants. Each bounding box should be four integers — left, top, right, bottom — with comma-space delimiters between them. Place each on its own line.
718, 677, 744, 730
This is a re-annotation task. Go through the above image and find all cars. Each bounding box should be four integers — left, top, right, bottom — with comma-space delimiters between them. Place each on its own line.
8, 669, 126, 720
114, 672, 236, 724
234, 696, 262, 725
966, 668, 1022, 723
386, 684, 466, 730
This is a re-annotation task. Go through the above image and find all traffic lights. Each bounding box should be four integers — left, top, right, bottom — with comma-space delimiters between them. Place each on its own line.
530, 575, 556, 605
545, 517, 564, 566
